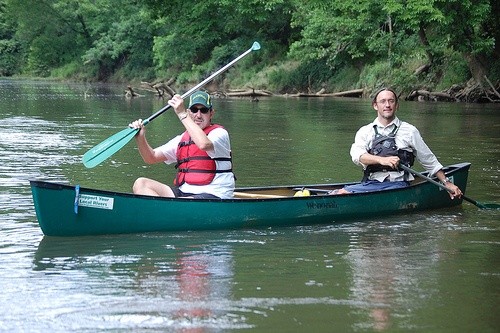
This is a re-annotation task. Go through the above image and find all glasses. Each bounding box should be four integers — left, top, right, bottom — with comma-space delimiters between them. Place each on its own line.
190, 106, 212, 113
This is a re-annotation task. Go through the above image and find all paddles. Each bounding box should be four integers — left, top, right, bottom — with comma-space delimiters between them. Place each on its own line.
398, 161, 500, 215
81, 43, 261, 168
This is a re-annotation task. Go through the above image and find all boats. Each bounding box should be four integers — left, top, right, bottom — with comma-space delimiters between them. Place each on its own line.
31, 162, 472, 238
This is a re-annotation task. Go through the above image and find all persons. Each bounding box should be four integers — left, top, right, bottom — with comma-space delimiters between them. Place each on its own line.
129, 91, 236, 199
332, 86, 463, 199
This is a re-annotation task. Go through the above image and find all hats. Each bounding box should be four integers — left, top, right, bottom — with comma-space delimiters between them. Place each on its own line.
188, 90, 212, 109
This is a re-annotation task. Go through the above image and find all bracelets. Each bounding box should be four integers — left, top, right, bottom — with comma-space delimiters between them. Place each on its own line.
443, 176, 451, 184
179, 112, 188, 119
